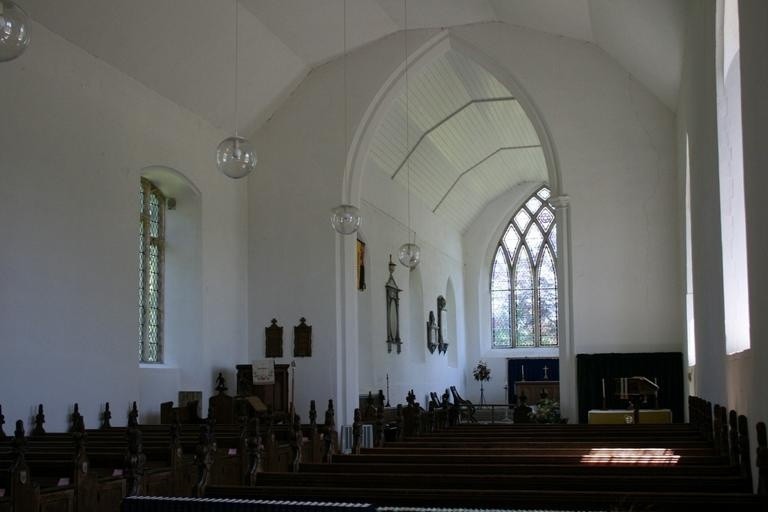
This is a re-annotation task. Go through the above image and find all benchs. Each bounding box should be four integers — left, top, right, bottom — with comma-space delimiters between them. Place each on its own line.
0, 391, 768, 512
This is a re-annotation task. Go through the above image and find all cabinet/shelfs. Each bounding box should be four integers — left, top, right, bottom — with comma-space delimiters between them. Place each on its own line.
236, 364, 289, 417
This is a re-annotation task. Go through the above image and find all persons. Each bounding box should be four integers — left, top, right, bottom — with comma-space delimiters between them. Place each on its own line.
216, 372, 224, 387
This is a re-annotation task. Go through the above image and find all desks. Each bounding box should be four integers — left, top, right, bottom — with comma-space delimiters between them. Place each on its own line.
515, 381, 559, 406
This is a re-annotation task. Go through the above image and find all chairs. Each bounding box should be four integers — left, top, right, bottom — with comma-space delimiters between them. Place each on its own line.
451, 387, 477, 423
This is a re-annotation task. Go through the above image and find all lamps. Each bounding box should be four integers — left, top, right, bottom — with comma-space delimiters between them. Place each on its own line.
0, 0, 32, 63
217, 0, 258, 178
330, 0, 361, 234
398, 0, 420, 268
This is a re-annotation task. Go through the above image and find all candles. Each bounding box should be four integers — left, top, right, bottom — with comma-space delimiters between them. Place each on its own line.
621, 378, 624, 397
625, 378, 628, 395
602, 378, 605, 399
522, 365, 524, 376
545, 365, 547, 377
